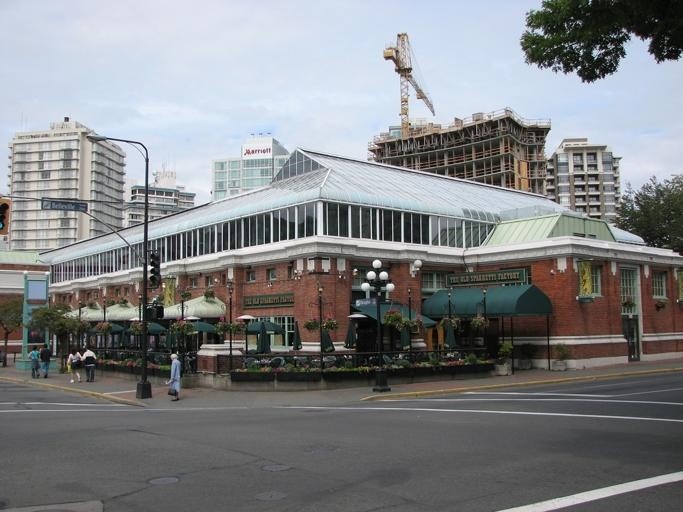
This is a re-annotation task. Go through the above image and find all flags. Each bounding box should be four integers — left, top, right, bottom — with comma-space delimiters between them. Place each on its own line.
576, 259, 594, 303
163, 276, 176, 309
678, 269, 683, 302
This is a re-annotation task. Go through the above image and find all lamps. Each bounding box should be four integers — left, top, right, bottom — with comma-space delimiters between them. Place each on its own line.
411, 259, 422, 278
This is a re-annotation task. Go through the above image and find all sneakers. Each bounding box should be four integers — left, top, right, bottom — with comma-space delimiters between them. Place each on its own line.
87, 379, 94, 382
70, 379, 81, 384
32, 373, 48, 379
171, 398, 180, 401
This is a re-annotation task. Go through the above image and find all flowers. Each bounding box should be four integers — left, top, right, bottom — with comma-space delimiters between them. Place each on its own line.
169, 316, 195, 334
179, 286, 191, 298
383, 308, 402, 327
215, 316, 230, 332
323, 312, 339, 331
95, 322, 113, 336
130, 321, 148, 336
230, 322, 247, 335
304, 316, 320, 333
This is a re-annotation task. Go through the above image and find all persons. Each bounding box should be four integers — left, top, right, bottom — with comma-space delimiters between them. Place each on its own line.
28, 346, 40, 378
66, 346, 83, 384
81, 346, 96, 382
164, 354, 182, 402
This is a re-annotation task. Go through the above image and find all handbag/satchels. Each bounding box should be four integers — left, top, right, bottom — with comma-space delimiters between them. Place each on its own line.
167, 383, 177, 396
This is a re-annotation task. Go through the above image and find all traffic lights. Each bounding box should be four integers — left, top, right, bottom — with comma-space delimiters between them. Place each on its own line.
0, 203, 9, 230
150, 253, 160, 283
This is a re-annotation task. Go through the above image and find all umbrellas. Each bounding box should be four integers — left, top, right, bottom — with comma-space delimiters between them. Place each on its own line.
257, 321, 272, 353
292, 320, 303, 352
190, 320, 219, 334
124, 322, 168, 337
242, 320, 286, 335
400, 325, 410, 350
321, 327, 335, 353
444, 317, 457, 347
344, 319, 357, 349
87, 322, 123, 337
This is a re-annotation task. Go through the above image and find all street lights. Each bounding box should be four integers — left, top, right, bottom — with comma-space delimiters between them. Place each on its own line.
86, 135, 152, 399
483, 289, 487, 321
181, 297, 184, 319
138, 294, 142, 322
226, 281, 233, 371
78, 300, 82, 321
361, 259, 395, 392
47, 294, 51, 307
103, 298, 106, 322
448, 293, 451, 318
318, 286, 324, 352
408, 288, 411, 321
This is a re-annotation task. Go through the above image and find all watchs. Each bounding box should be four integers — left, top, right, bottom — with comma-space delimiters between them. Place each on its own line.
40, 344, 52, 378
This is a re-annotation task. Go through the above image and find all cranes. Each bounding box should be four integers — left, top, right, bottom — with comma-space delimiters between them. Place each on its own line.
383, 31, 435, 138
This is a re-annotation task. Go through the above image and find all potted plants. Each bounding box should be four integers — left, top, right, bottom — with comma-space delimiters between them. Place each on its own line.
229, 340, 514, 381
95, 351, 183, 378
440, 317, 462, 329
519, 344, 538, 370
622, 300, 636, 313
88, 298, 97, 309
203, 286, 216, 302
470, 316, 490, 329
551, 344, 571, 372
105, 297, 115, 307
396, 318, 418, 332
656, 300, 667, 309
118, 297, 128, 305
